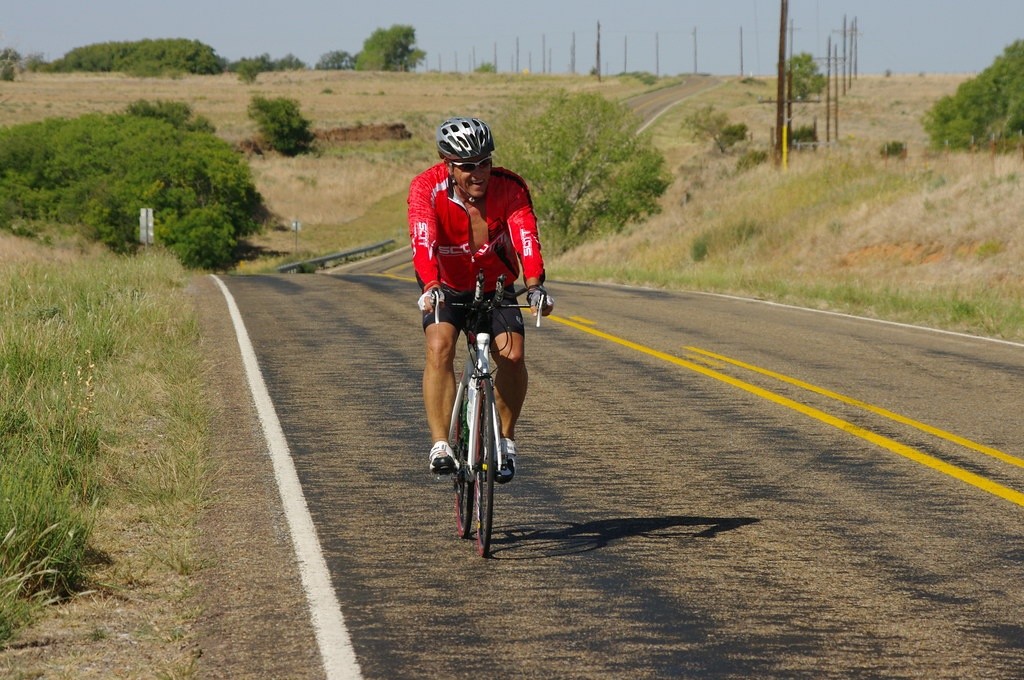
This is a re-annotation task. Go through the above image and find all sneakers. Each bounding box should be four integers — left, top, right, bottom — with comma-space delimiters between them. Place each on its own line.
428, 440, 461, 471
493, 437, 517, 484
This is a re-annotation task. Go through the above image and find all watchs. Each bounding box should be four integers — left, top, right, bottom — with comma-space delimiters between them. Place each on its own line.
528, 284, 546, 292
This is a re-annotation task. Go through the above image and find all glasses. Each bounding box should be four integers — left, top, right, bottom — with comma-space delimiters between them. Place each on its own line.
451, 152, 493, 171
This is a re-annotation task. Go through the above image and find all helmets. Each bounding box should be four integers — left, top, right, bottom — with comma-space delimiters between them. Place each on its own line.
436, 117, 495, 159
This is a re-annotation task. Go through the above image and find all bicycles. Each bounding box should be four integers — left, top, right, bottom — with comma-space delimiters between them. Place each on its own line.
427, 286, 547, 558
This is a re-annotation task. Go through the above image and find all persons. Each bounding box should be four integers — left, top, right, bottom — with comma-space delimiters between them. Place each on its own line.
408, 118, 553, 484
